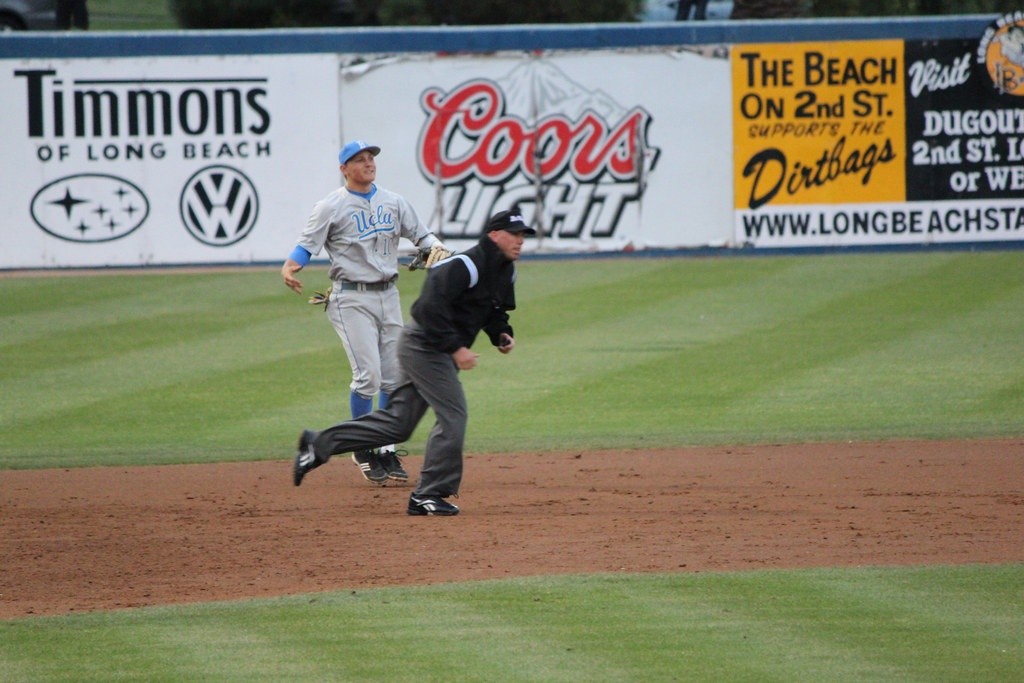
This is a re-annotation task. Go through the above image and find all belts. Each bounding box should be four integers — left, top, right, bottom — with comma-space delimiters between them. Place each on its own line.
342, 282, 388, 291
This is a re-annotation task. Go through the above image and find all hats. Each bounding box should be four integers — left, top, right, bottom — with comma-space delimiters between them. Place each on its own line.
339, 141, 380, 165
485, 210, 536, 235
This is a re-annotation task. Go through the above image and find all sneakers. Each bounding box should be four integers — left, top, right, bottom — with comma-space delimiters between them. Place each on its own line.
293, 430, 322, 486
407, 492, 459, 516
351, 450, 409, 482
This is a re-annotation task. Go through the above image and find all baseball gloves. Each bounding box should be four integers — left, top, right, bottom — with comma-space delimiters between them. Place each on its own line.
407, 242, 457, 275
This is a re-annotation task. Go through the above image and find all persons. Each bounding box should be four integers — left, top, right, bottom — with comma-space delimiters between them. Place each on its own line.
282, 141, 455, 484
293, 210, 538, 515
